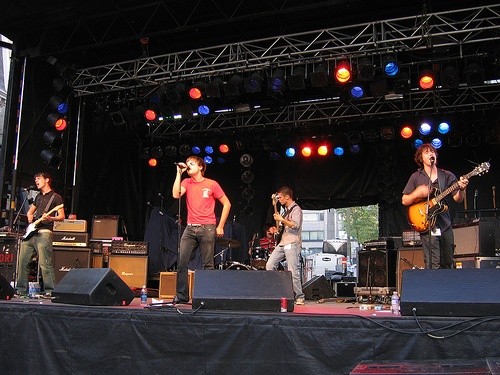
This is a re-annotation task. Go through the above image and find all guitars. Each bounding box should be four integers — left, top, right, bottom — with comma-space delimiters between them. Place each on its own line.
404, 161, 491, 234
270, 192, 284, 248
20, 203, 65, 242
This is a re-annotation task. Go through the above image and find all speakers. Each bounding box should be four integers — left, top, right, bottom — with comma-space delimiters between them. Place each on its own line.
400, 268, 500, 317
50, 267, 136, 306
53, 247, 90, 287
357, 250, 397, 287
91, 215, 122, 239
0, 273, 15, 300
192, 270, 294, 312
108, 255, 148, 289
302, 275, 336, 299
397, 247, 424, 296
452, 225, 480, 256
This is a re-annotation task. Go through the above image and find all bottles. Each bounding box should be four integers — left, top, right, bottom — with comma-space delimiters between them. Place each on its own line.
28, 282, 40, 297
141, 285, 147, 305
392, 291, 399, 315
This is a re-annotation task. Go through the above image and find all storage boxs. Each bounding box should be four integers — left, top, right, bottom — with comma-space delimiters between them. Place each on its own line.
52, 219, 91, 287
159, 271, 194, 299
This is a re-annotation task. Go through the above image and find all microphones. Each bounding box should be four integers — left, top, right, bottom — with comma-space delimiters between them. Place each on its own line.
24, 185, 35, 191
430, 157, 435, 164
174, 162, 190, 170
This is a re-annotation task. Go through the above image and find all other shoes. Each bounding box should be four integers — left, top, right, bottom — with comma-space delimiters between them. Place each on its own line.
43, 293, 51, 299
296, 298, 305, 305
174, 296, 187, 303
13, 294, 29, 299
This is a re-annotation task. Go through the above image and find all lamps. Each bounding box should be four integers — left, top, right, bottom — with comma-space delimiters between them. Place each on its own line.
95, 44, 500, 166
237, 166, 255, 216
41, 94, 66, 164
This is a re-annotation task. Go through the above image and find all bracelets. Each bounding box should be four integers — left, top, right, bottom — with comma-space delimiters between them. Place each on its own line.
459, 188, 465, 191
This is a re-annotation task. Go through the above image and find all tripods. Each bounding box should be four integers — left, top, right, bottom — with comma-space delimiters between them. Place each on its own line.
150, 169, 192, 314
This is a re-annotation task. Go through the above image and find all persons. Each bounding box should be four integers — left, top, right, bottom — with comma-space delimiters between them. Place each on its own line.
172, 155, 231, 303
265, 186, 305, 305
15, 173, 65, 298
401, 143, 469, 268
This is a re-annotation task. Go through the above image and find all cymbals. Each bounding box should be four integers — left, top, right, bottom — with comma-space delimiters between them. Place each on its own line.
216, 238, 242, 249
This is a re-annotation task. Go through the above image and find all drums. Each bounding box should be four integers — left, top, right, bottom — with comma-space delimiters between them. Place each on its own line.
250, 246, 268, 268
225, 263, 258, 271
